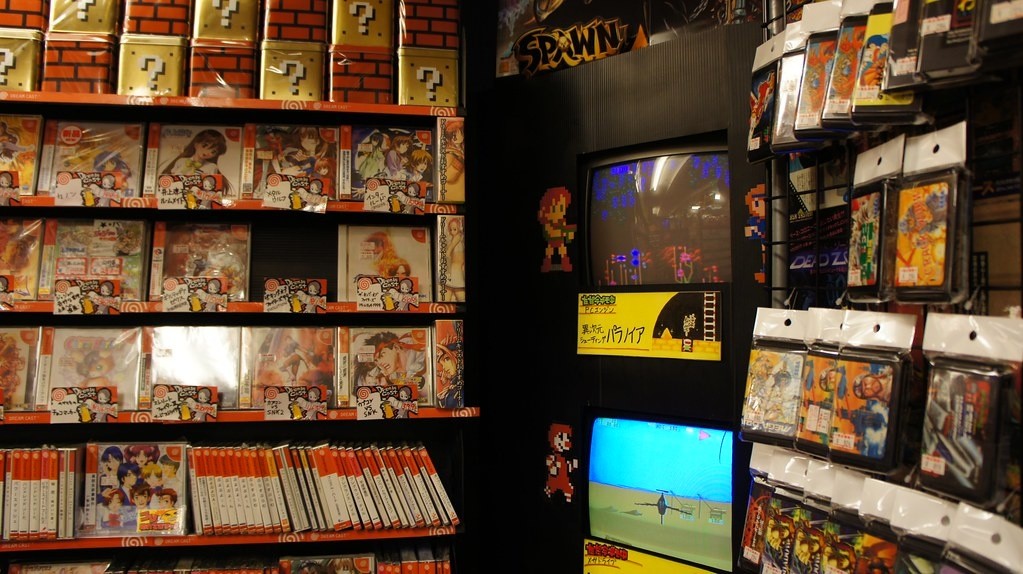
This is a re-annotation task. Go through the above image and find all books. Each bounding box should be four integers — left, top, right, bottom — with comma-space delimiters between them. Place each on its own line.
0, 115, 464, 574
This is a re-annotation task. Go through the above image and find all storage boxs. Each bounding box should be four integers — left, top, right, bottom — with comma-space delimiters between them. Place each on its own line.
0, 1, 461, 106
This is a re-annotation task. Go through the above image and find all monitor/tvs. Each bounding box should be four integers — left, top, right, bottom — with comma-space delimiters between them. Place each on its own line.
576, 139, 734, 292
579, 403, 756, 574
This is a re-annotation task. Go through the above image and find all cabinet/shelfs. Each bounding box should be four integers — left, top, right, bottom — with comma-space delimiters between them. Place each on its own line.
0, 92, 482, 573
